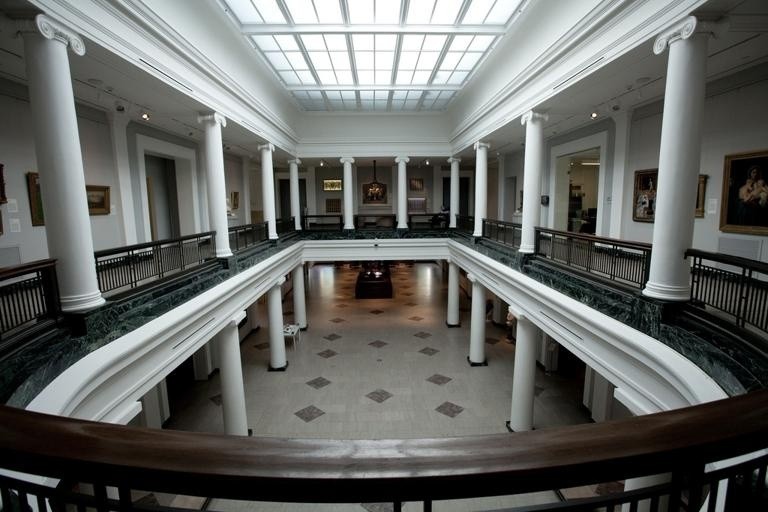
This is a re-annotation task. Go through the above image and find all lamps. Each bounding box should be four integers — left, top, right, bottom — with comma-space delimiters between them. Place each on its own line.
580, 160, 600, 166
367, 161, 383, 201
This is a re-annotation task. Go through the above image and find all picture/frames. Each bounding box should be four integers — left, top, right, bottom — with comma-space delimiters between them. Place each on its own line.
86, 184, 110, 215
409, 178, 424, 191
323, 178, 344, 192
718, 149, 768, 236
633, 168, 658, 223
25, 172, 44, 226
694, 174, 709, 217
231, 191, 240, 209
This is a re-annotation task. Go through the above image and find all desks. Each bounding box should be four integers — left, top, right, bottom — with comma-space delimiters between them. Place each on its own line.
283, 324, 301, 352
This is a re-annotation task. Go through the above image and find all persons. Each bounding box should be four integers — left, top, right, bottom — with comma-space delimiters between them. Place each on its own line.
745, 179, 767, 202
747, 165, 759, 193
648, 178, 653, 192
739, 178, 753, 200
428, 205, 449, 229
